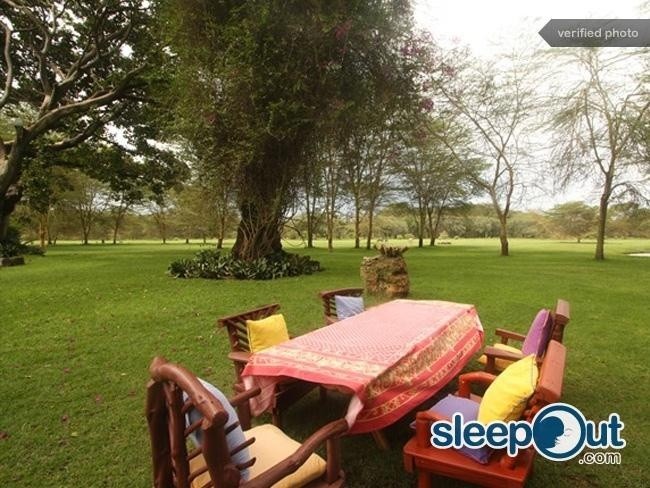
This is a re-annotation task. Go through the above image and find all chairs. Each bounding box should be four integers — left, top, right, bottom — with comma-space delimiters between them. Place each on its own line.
144, 285, 572, 488
